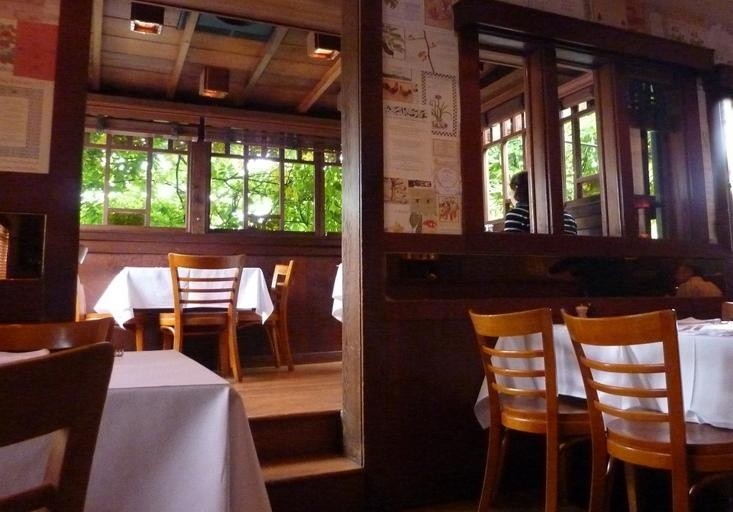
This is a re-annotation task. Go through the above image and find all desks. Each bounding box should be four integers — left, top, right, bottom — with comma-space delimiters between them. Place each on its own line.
1, 347, 273, 512
474, 314, 733, 452
93, 266, 274, 333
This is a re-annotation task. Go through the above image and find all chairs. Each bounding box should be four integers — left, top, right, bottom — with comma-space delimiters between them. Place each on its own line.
560, 306, 733, 511
1, 339, 114, 510
0, 315, 113, 355
238, 259, 295, 371
466, 305, 589, 511
154, 252, 245, 383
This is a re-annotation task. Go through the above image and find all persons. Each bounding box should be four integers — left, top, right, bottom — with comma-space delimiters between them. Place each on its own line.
503, 170, 577, 234
